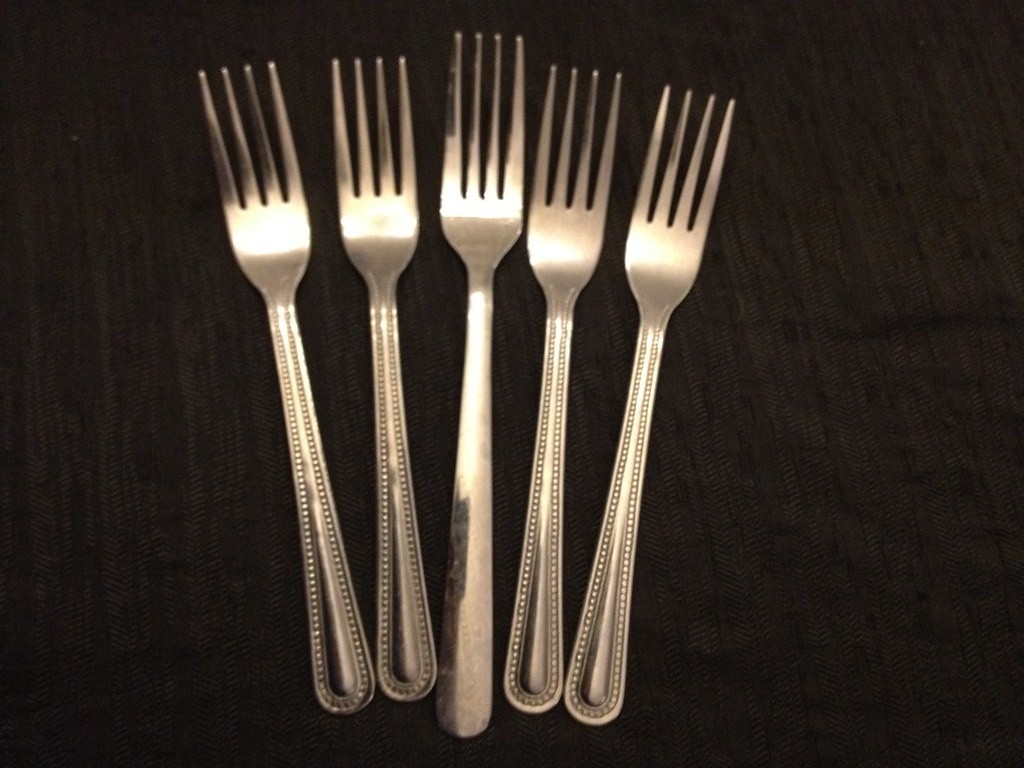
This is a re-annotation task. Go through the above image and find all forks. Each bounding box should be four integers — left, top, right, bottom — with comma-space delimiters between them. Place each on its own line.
196, 61, 376, 717
331, 56, 438, 703
564, 84, 737, 726
502, 64, 624, 718
435, 29, 525, 740
